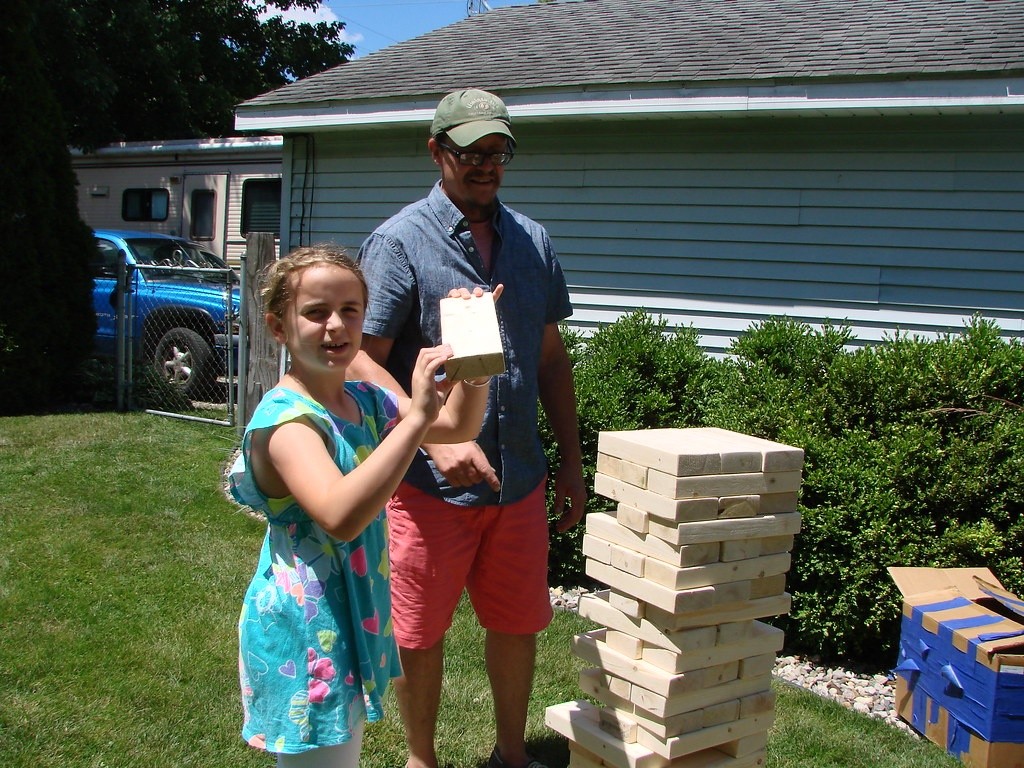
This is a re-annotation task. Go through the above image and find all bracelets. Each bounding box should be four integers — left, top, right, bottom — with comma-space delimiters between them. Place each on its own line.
463, 375, 494, 388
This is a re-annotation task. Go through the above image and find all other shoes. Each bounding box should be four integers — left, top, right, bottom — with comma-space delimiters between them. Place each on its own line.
489, 750, 548, 768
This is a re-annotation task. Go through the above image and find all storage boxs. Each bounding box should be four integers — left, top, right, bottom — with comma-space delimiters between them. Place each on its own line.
887, 566, 1024, 768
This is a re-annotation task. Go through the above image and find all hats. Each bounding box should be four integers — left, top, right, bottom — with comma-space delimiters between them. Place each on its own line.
430, 90, 517, 149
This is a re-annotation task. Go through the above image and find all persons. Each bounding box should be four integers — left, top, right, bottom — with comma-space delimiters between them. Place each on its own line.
354, 87, 587, 768
223, 241, 505, 768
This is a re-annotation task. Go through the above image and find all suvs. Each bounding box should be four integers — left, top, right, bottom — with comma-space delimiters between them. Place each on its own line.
88, 226, 245, 407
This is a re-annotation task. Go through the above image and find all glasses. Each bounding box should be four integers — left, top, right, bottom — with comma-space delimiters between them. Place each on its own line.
439, 139, 515, 166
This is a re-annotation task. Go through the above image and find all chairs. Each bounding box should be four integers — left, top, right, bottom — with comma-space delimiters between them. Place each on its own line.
154, 246, 178, 275
